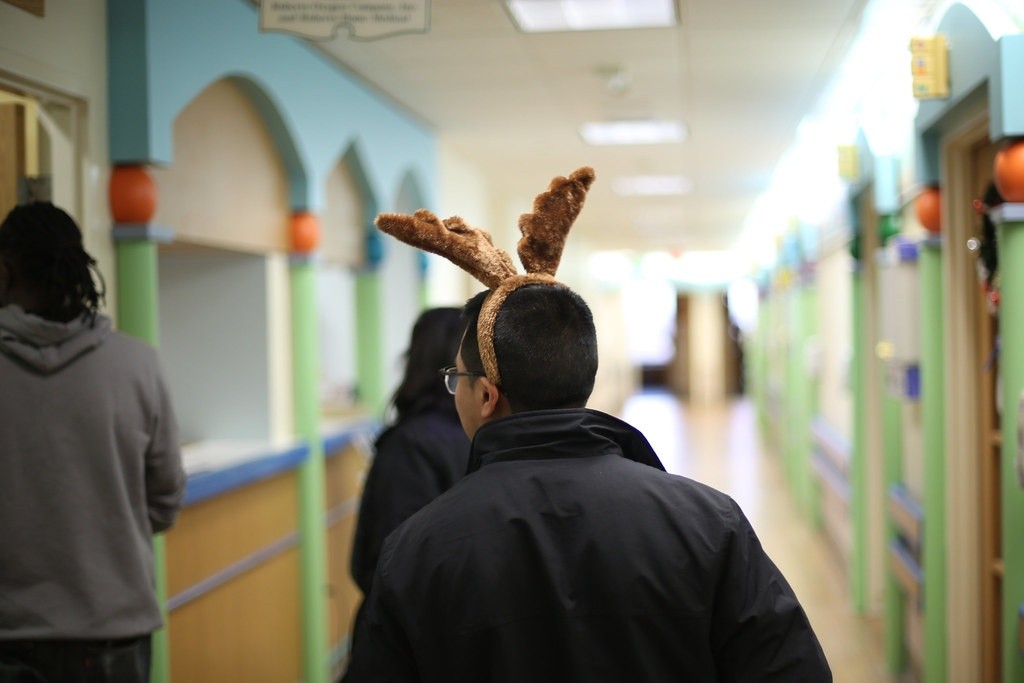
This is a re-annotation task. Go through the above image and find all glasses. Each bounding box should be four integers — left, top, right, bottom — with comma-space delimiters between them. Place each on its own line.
439, 365, 487, 394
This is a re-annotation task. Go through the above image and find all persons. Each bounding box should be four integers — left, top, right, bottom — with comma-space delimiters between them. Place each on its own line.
337, 284, 831, 683
0, 202, 185, 683
349, 308, 471, 597
